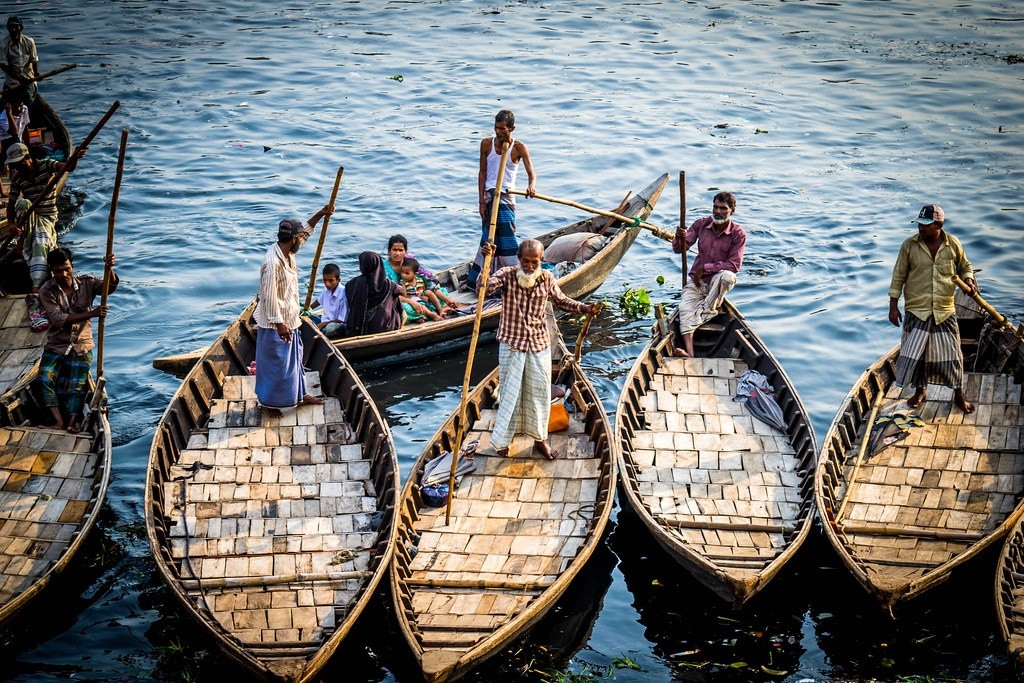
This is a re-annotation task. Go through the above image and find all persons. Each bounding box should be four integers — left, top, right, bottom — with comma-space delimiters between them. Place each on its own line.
38, 247, 120, 434
382, 234, 459, 324
310, 263, 350, 336
477, 239, 602, 460
672, 192, 746, 356
465, 110, 536, 297
253, 204, 335, 419
345, 251, 408, 336
887, 204, 978, 413
4, 142, 89, 293
0, 16, 43, 171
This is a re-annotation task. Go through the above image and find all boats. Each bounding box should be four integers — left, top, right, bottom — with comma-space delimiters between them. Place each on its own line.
813, 269, 1023, 620
0, 289, 74, 410
0, 83, 74, 243
0, 348, 112, 621
142, 291, 401, 683
150, 169, 674, 382
993, 510, 1024, 669
386, 300, 617, 683
612, 294, 820, 612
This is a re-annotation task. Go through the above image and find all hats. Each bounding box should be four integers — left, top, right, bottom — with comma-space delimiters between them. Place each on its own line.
278, 218, 305, 236
911, 204, 945, 225
6, 16, 22, 24
3, 142, 29, 164
